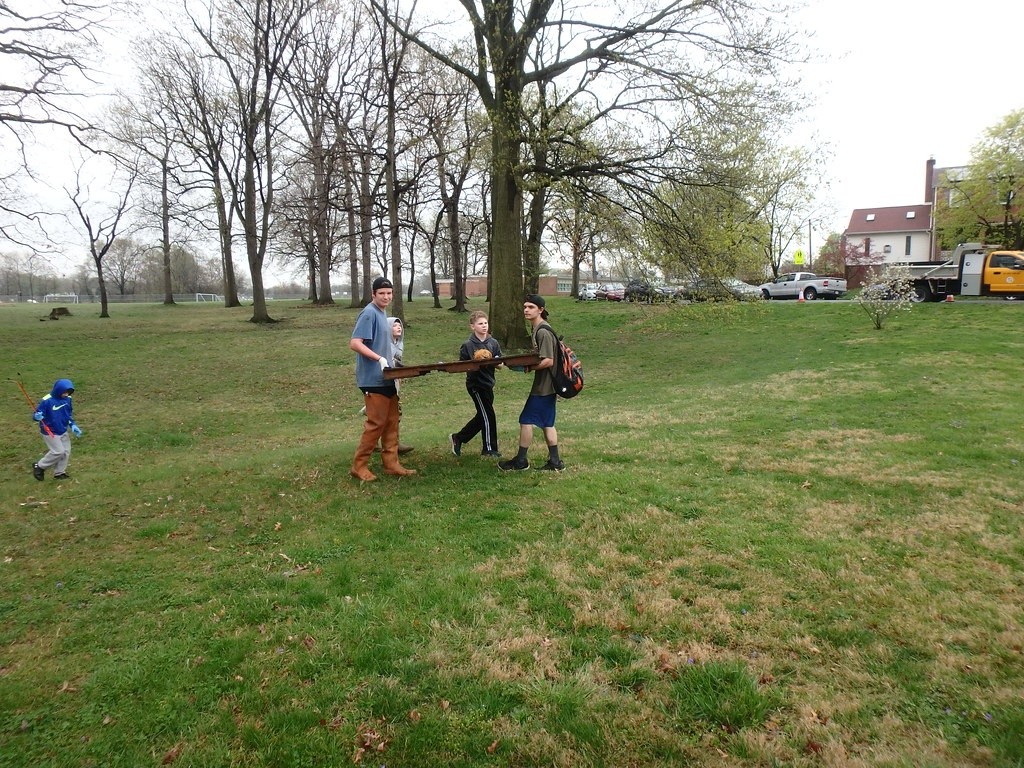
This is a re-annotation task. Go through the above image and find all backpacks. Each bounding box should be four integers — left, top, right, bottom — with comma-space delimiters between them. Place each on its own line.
535, 325, 584, 398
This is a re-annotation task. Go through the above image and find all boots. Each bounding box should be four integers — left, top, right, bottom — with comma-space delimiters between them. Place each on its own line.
380, 395, 417, 476
351, 392, 390, 481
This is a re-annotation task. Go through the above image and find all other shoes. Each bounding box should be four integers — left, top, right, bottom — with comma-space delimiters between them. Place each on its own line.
374, 444, 382, 452
398, 442, 414, 453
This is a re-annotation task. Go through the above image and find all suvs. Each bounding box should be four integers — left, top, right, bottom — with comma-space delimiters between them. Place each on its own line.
624, 277, 675, 302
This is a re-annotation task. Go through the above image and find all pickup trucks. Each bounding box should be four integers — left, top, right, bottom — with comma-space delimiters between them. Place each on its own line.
758, 272, 847, 299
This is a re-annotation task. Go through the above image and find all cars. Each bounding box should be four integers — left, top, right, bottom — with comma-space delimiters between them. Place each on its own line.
860, 278, 887, 299
578, 283, 602, 301
28, 300, 38, 303
420, 290, 430, 296
595, 281, 626, 301
673, 277, 763, 303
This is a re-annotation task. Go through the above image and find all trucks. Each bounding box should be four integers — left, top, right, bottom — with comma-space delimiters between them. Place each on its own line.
885, 242, 1024, 303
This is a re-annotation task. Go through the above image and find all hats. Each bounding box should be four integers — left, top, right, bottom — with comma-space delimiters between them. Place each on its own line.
524, 294, 549, 319
373, 277, 392, 290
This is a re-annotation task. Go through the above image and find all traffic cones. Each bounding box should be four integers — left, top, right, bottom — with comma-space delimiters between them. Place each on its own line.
796, 288, 807, 303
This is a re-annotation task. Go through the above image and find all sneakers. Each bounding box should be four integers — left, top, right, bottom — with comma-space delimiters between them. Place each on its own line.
54, 473, 72, 481
32, 462, 44, 481
480, 450, 503, 458
449, 433, 461, 457
496, 456, 530, 473
533, 459, 566, 473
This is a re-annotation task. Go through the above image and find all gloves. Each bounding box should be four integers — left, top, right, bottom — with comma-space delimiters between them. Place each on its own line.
378, 357, 389, 372
71, 425, 82, 438
34, 411, 44, 421
394, 358, 404, 367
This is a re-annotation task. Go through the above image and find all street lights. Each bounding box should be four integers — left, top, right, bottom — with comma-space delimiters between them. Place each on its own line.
809, 222, 816, 273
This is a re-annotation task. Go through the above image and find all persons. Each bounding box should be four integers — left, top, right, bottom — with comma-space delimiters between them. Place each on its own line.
373, 317, 414, 455
497, 294, 566, 472
449, 311, 503, 458
349, 276, 417, 482
32, 379, 82, 481
